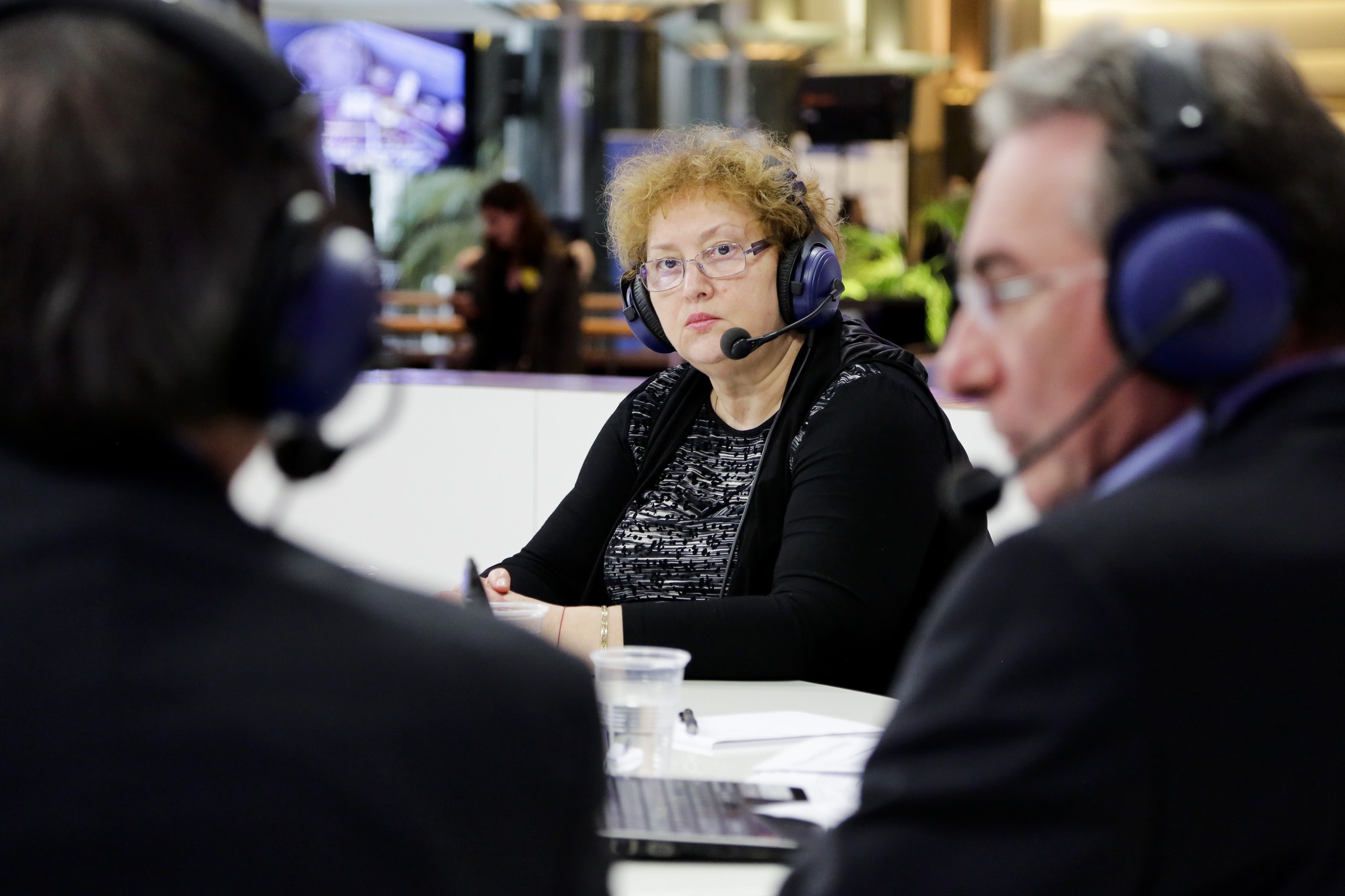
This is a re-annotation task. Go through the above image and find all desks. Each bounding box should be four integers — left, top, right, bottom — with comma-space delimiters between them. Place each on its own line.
599, 680, 899, 896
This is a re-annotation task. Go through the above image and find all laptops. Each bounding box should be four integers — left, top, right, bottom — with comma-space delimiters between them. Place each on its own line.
461, 558, 828, 863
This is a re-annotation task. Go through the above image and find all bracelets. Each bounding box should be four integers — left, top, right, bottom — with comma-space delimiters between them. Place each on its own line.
601, 605, 608, 648
555, 605, 566, 653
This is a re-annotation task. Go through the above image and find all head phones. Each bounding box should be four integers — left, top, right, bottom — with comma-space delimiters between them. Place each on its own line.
619, 152, 842, 355
1113, 27, 1291, 386
0, 0, 385, 418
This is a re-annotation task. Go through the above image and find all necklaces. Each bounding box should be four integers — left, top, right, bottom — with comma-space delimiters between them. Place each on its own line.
714, 395, 718, 413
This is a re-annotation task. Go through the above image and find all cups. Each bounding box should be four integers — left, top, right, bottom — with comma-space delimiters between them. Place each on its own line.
588, 644, 692, 761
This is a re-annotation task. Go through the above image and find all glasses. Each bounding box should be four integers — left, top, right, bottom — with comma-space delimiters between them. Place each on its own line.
636, 235, 776, 292
957, 265, 1113, 328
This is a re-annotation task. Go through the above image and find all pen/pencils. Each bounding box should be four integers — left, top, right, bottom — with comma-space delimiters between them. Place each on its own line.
679, 707, 699, 734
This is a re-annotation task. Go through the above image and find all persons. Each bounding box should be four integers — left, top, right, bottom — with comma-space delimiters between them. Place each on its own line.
447, 178, 587, 374
430, 120, 1003, 696
772, 13, 1345, 896
0, 0, 608, 896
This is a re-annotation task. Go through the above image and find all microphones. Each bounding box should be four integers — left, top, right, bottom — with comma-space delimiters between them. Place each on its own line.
939, 282, 1225, 511
720, 280, 847, 358
